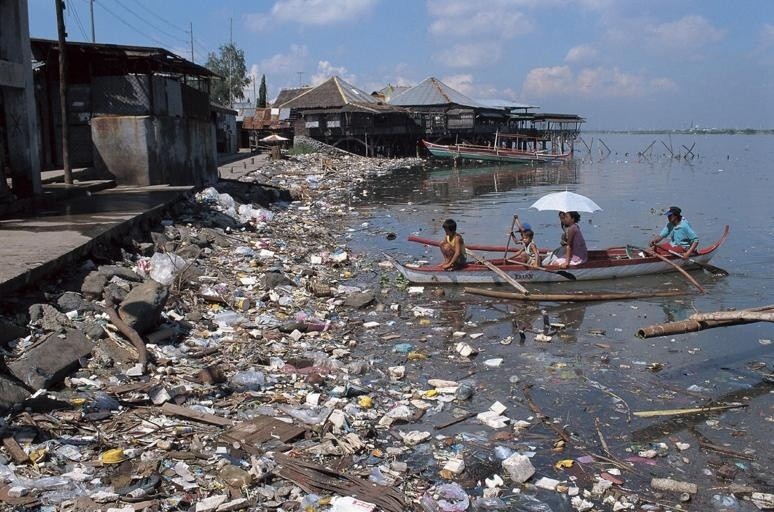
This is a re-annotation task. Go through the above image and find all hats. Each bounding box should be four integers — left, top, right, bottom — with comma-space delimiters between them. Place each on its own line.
516, 222, 531, 232
664, 206, 681, 216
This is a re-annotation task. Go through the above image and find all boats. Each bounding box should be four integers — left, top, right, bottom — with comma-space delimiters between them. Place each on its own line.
422, 139, 569, 164
382, 224, 730, 283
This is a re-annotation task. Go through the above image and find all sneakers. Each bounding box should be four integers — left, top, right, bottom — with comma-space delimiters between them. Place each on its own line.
626, 246, 635, 259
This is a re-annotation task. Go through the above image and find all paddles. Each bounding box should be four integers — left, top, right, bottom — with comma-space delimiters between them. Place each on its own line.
503, 256, 576, 281
649, 241, 728, 277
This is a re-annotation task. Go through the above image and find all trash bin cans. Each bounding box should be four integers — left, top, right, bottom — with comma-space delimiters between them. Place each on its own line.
271, 146, 280, 162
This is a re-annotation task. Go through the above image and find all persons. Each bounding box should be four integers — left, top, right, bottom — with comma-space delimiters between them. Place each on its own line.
437, 218, 467, 272
650, 206, 700, 261
502, 229, 540, 268
547, 210, 588, 270
539, 211, 569, 265
511, 222, 535, 248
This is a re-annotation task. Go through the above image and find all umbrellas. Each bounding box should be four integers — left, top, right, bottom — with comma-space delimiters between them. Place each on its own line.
527, 187, 604, 227
256, 134, 293, 142
